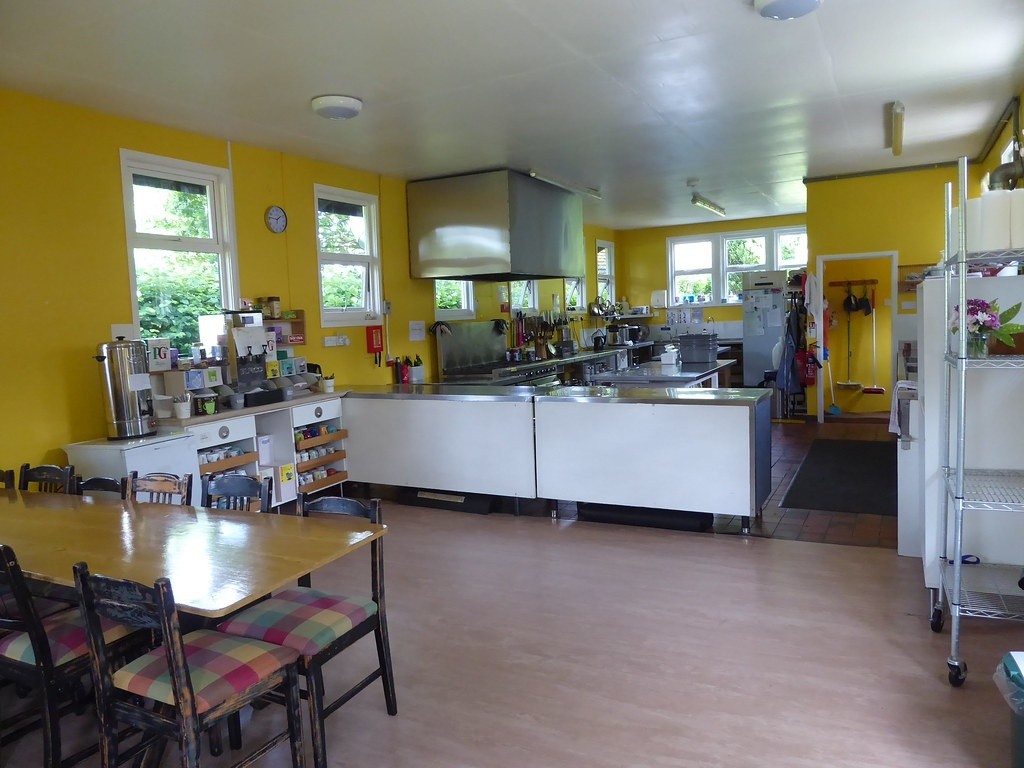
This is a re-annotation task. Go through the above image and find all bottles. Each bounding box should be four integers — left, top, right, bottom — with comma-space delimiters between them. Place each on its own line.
395, 355, 422, 384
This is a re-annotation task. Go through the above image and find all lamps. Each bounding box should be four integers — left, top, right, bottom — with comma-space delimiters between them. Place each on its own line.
530, 169, 602, 201
892, 100, 904, 155
755, 0, 820, 20
310, 96, 362, 121
691, 195, 729, 218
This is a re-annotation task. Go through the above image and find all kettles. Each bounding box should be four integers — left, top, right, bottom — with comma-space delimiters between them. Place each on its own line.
593, 335, 606, 353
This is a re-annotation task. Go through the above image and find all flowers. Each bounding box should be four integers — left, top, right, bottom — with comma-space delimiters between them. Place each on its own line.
948, 296, 1024, 348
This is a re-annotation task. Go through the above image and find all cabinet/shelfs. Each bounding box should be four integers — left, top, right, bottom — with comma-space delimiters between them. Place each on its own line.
930, 157, 1024, 685
62, 397, 350, 515
263, 308, 306, 345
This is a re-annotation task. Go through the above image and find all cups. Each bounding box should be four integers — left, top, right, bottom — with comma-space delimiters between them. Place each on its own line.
212, 345, 222, 362
203, 401, 215, 414
321, 379, 335, 394
190, 346, 201, 365
198, 445, 248, 510
169, 348, 178, 367
173, 402, 191, 418
295, 425, 337, 485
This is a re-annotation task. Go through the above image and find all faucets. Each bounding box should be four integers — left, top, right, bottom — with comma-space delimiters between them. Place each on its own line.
706, 316, 715, 334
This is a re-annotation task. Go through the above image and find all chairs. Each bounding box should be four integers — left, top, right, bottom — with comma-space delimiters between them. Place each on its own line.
0, 463, 398, 767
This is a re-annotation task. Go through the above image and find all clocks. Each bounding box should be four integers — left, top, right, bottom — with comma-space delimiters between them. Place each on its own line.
263, 206, 287, 233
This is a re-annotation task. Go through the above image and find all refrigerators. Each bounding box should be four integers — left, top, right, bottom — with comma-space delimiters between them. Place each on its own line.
742, 270, 787, 387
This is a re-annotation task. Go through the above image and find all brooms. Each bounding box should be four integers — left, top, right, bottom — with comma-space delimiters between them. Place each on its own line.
827, 355, 842, 416
862, 289, 886, 395
836, 286, 863, 391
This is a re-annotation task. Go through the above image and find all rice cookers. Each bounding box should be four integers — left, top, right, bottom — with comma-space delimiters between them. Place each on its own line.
606, 323, 629, 346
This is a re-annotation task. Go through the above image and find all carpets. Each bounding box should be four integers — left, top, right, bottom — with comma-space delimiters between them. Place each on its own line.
779, 436, 898, 516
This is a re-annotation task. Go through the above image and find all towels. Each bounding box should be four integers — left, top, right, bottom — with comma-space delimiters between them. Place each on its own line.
888, 379, 919, 436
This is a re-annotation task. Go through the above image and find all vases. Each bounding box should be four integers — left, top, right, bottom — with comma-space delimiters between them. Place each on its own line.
968, 332, 987, 358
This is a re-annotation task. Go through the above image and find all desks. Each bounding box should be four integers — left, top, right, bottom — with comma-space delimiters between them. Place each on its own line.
588, 360, 737, 387
1, 487, 386, 749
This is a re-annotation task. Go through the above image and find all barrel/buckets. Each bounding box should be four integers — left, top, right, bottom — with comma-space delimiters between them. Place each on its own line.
92, 336, 158, 438
